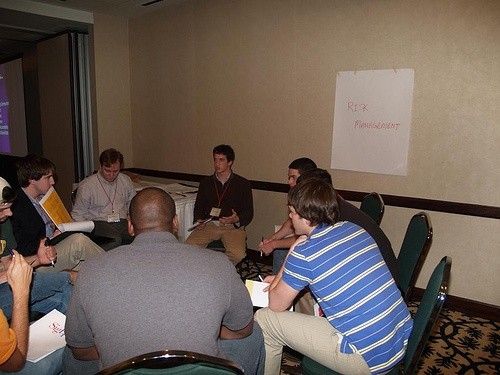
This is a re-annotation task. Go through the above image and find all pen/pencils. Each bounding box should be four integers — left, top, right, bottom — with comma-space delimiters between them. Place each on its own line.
259, 274, 265, 282
45, 241, 55, 267
204, 218, 211, 224
260, 236, 264, 259
7, 246, 14, 257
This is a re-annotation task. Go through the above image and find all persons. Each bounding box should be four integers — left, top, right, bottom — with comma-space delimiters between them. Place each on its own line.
183, 144, 254, 267
254, 177, 414, 375
0, 153, 106, 375
260, 157, 400, 288
70, 148, 138, 251
64, 187, 266, 375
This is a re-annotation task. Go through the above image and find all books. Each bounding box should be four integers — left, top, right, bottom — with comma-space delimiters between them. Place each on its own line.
25, 309, 68, 363
39, 186, 95, 233
245, 279, 293, 311
187, 220, 204, 231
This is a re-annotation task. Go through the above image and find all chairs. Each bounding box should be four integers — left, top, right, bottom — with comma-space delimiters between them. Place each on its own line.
72, 188, 453, 375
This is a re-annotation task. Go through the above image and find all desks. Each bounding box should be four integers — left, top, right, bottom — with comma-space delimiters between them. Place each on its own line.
133, 179, 197, 244
